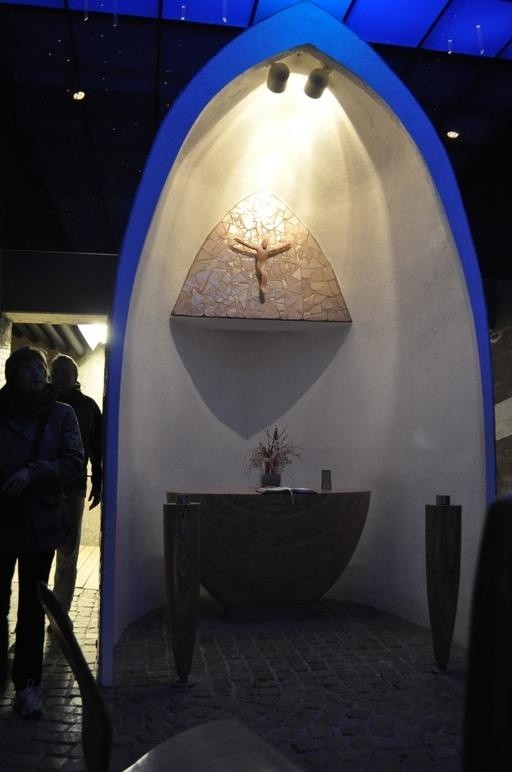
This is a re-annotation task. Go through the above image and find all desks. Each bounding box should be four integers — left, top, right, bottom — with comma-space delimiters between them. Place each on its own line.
162, 486, 373, 621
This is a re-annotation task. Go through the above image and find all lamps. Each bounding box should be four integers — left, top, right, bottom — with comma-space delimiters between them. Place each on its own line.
265, 61, 331, 100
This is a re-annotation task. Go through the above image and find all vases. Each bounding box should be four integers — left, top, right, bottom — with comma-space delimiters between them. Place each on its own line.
261, 472, 281, 485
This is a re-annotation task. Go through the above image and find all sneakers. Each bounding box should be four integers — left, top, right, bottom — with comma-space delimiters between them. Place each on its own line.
0, 620, 72, 717
162, 681, 192, 713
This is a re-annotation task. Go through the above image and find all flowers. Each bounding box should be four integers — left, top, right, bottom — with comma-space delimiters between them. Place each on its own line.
245, 425, 304, 474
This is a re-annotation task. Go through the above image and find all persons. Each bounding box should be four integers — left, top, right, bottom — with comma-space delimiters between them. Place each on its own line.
1, 343, 89, 721
43, 351, 104, 634
231, 235, 295, 297
